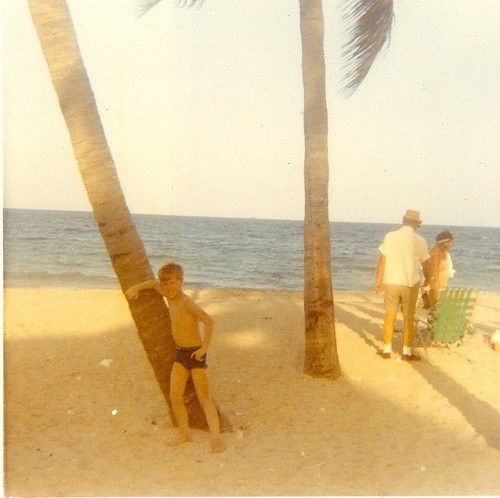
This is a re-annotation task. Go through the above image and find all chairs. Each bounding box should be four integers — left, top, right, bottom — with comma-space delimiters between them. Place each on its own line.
414, 284, 484, 363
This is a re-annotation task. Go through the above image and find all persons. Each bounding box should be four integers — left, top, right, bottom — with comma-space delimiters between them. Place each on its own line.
423, 229, 454, 329
374, 210, 431, 361
126, 261, 227, 453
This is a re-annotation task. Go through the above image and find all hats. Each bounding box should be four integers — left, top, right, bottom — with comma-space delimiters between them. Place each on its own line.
404, 210, 421, 224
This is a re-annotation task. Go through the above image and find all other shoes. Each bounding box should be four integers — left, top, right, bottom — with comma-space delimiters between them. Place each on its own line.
381, 351, 390, 358
401, 353, 421, 361
